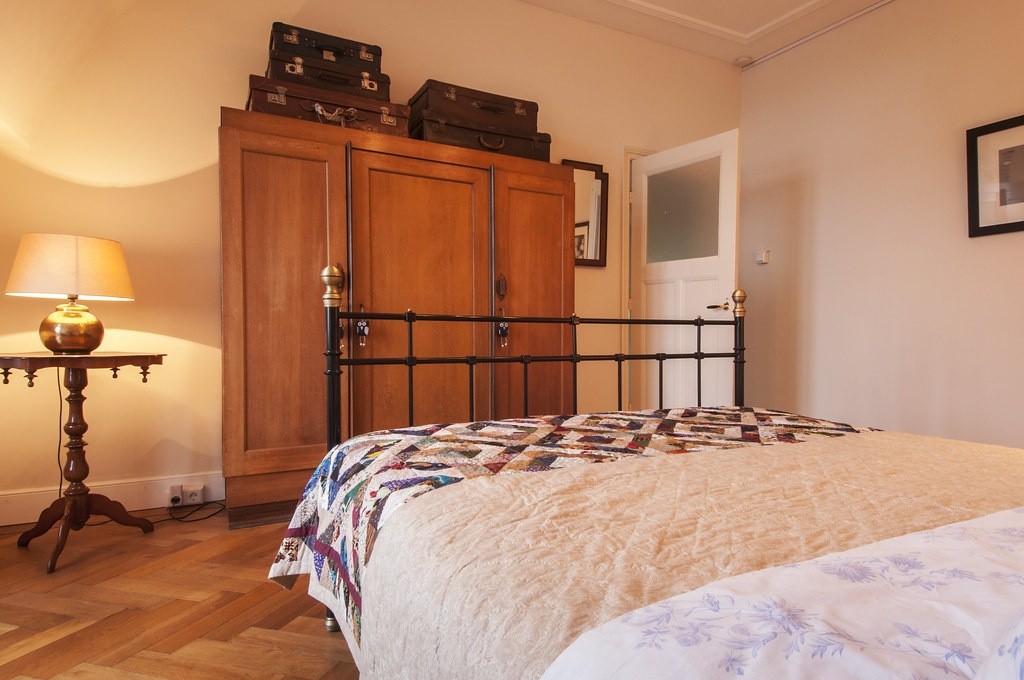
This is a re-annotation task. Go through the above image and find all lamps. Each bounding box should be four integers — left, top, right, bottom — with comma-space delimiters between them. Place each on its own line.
4, 232, 135, 356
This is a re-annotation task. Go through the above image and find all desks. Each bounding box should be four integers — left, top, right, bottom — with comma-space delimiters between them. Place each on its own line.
0, 351, 168, 574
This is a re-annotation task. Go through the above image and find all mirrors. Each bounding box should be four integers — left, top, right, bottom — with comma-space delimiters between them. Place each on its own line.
562, 158, 608, 267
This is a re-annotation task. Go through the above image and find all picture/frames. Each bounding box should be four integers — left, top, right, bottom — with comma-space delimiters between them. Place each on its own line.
966, 114, 1024, 237
574, 221, 589, 259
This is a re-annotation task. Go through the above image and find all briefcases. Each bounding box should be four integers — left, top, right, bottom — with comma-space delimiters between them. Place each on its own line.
407, 79, 552, 162
246, 22, 412, 138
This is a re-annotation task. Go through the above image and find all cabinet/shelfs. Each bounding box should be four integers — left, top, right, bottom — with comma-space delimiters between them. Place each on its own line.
218, 106, 575, 530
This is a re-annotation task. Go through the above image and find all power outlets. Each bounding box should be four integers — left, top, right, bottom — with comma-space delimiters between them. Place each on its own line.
165, 485, 182, 508
182, 484, 204, 505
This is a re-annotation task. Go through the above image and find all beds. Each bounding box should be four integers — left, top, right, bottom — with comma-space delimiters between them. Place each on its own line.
265, 263, 1023, 680
